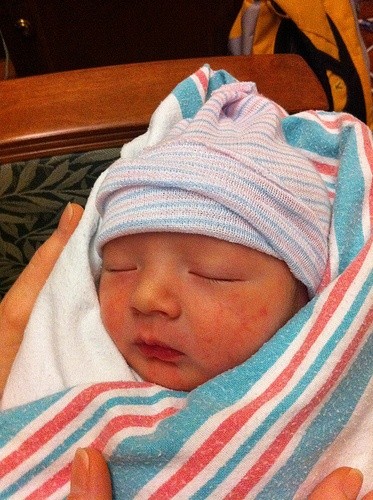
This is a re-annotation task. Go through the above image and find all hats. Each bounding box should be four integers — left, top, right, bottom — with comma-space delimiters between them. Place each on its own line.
94, 80, 330, 300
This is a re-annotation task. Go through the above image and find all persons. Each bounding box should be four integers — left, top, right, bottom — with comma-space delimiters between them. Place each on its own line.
0, 63, 373, 500
